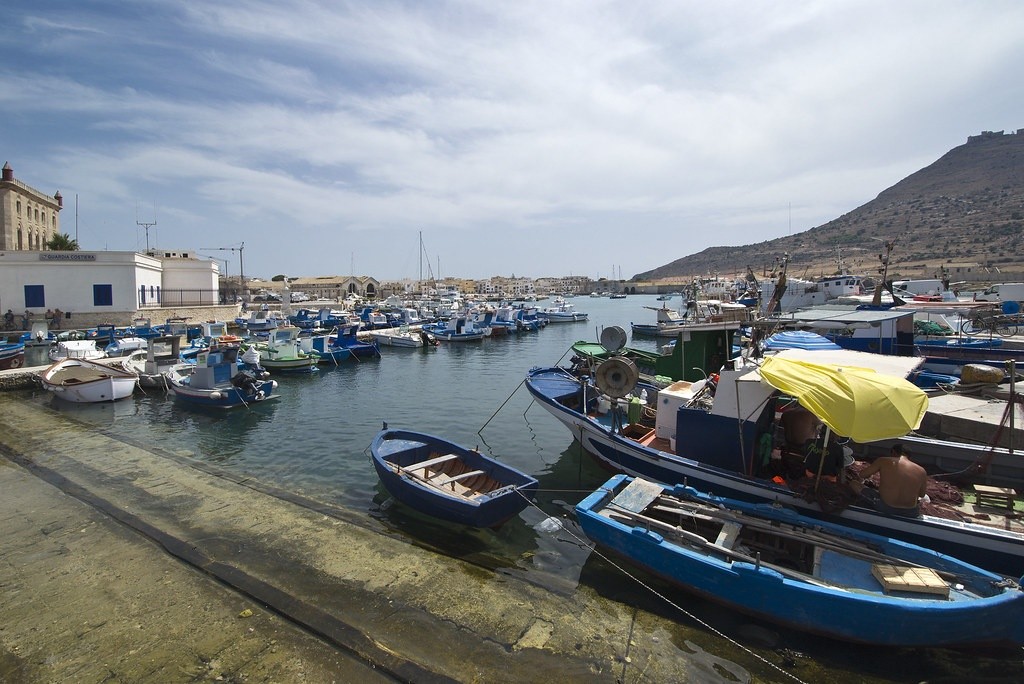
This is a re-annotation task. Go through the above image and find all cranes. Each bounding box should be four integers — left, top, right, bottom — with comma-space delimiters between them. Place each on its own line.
197, 242, 246, 278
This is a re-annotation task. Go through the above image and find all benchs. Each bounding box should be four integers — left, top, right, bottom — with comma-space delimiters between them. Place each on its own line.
712, 520, 743, 555
596, 478, 666, 526
404, 455, 459, 473
437, 469, 483, 487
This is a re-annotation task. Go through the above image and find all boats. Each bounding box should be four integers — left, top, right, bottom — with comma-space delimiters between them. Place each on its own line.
372, 420, 539, 528
525, 238, 1024, 649
0, 337, 26, 360
371, 293, 550, 348
18, 308, 383, 409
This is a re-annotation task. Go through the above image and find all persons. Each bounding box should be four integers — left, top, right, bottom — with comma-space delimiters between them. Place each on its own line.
45, 309, 61, 330
953, 288, 959, 297
780, 403, 822, 446
4, 310, 14, 329
21, 310, 33, 330
848, 444, 927, 517
260, 301, 269, 311
801, 424, 844, 482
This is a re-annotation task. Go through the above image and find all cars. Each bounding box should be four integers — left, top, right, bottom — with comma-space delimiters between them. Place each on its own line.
220, 291, 308, 302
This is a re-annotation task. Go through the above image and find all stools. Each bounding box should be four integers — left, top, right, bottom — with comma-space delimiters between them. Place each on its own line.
971, 484, 1017, 512
